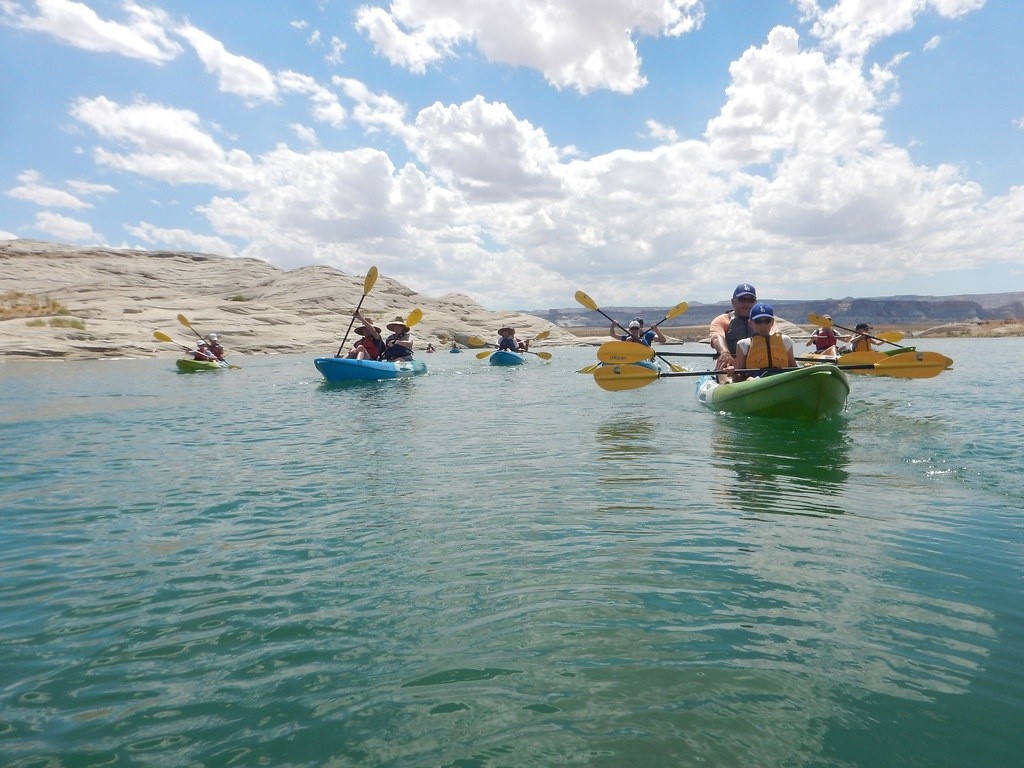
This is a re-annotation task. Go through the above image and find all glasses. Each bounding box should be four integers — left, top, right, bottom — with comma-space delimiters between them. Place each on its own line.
631, 327, 639, 330
733, 297, 757, 305
755, 318, 772, 324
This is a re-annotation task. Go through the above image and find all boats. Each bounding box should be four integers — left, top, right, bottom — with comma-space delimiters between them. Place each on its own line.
426, 349, 433, 353
313, 357, 427, 382
695, 364, 851, 421
451, 348, 461, 353
490, 350, 526, 365
802, 347, 953, 376
176, 358, 221, 373
602, 359, 661, 371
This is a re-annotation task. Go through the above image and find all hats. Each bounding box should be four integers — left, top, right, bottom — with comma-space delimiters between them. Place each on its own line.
209, 334, 217, 341
498, 326, 514, 336
386, 316, 411, 333
732, 283, 757, 298
354, 317, 381, 336
629, 321, 640, 328
855, 323, 874, 330
750, 303, 774, 320
633, 317, 643, 323
823, 315, 831, 319
197, 340, 206, 345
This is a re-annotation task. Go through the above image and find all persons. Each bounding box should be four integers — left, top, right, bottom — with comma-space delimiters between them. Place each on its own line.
341, 309, 382, 360
206, 334, 223, 361
723, 302, 817, 380
711, 284, 778, 385
805, 315, 852, 356
186, 340, 217, 362
626, 321, 650, 348
609, 317, 666, 346
427, 343, 434, 351
452, 341, 459, 349
495, 326, 519, 352
378, 317, 414, 362
850, 323, 887, 352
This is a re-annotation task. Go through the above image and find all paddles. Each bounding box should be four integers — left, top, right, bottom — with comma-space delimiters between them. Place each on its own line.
790, 331, 903, 342
446, 342, 463, 353
574, 290, 686, 373
807, 312, 904, 349
377, 308, 423, 360
336, 265, 379, 357
592, 351, 946, 393
468, 336, 552, 359
476, 330, 550, 359
177, 313, 229, 365
596, 339, 891, 374
154, 331, 242, 370
578, 302, 689, 374
